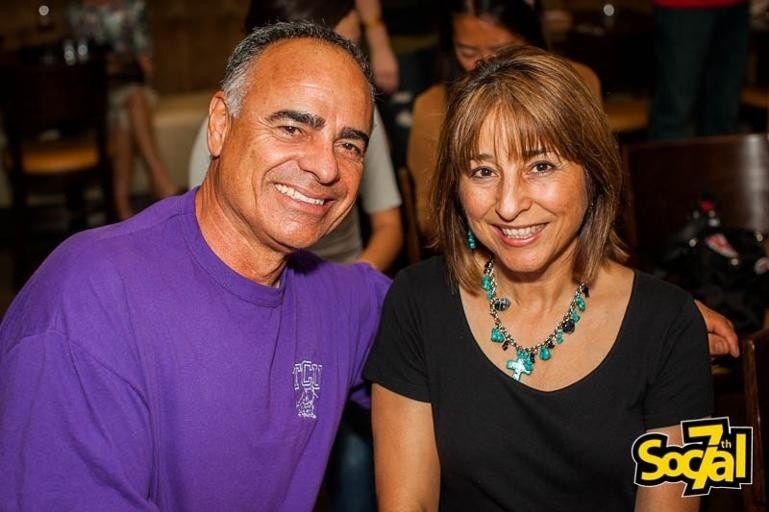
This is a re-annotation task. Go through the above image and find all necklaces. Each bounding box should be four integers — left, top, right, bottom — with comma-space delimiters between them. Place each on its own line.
478, 242, 590, 382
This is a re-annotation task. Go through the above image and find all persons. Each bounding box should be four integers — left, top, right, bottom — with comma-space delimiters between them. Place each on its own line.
358, 39, 714, 510
330, 2, 408, 95
0, 14, 742, 512
187, 43, 406, 512
403, 0, 548, 252
101, 2, 182, 223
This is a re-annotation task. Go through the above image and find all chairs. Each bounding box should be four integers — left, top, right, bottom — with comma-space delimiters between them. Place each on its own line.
2, 36, 121, 253
101, 0, 250, 198
356, 164, 423, 270
